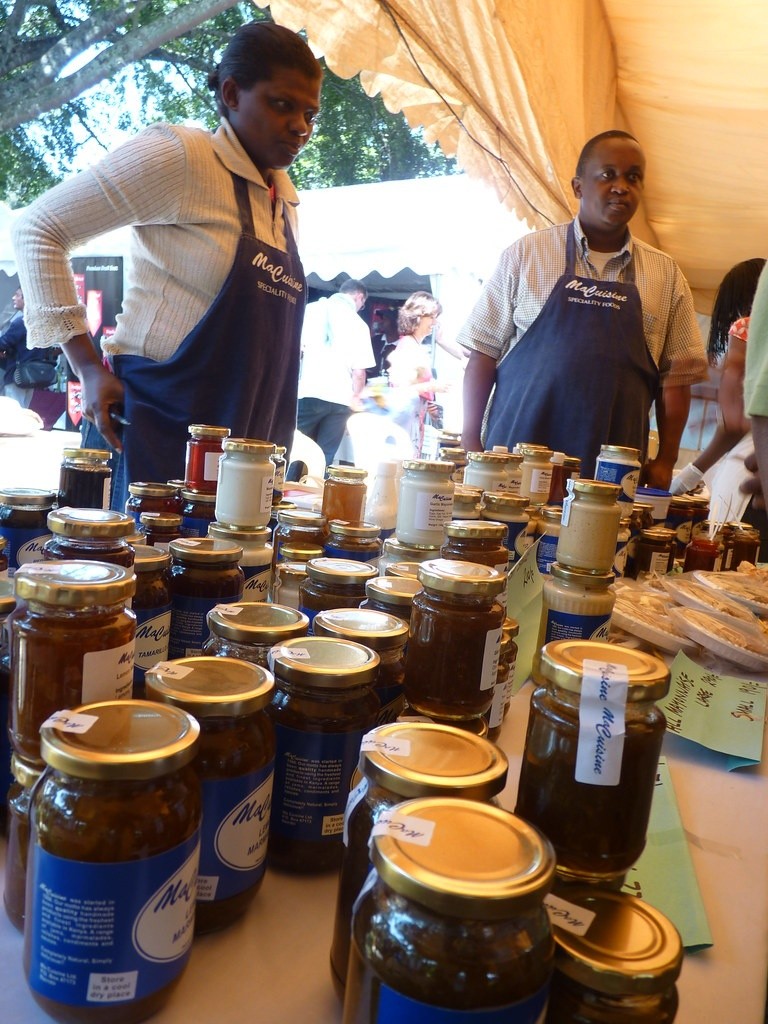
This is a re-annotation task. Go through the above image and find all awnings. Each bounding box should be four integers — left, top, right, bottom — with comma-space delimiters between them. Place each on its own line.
252, 1, 767, 317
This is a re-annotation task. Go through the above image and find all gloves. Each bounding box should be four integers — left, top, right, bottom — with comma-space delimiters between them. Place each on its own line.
667, 463, 703, 496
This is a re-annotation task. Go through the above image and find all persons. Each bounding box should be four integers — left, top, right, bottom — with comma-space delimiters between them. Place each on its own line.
457, 130, 710, 490
670, 258, 768, 523
0, 287, 34, 409
285, 279, 376, 483
386, 291, 452, 458
10, 21, 323, 514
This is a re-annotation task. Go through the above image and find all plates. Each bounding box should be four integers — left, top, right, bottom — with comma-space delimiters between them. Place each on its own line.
611, 570, 768, 674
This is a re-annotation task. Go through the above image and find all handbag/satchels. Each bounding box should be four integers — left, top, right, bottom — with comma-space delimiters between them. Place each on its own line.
13, 355, 59, 389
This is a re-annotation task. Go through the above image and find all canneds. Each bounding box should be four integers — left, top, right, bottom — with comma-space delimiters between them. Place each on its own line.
0, 422, 758, 1024
